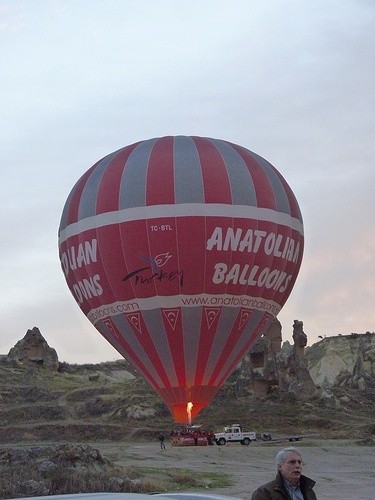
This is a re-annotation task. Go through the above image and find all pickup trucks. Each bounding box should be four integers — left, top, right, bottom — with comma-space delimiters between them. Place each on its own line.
214, 424, 256, 445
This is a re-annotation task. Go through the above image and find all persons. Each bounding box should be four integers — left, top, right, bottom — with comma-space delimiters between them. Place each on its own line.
169, 428, 216, 446
251, 448, 318, 500
158, 431, 166, 449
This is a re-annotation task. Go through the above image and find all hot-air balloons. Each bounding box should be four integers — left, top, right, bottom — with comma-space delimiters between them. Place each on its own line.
58, 136, 304, 447
